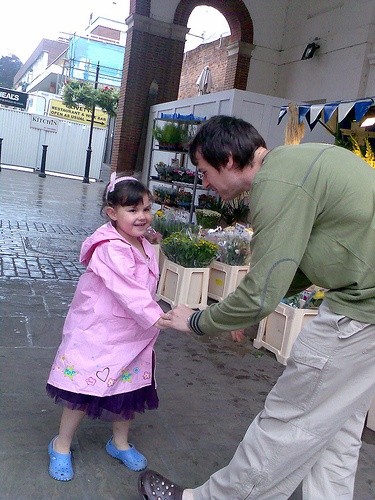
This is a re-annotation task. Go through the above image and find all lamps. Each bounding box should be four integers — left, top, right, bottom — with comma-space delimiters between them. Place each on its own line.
300, 36, 319, 60
359, 114, 375, 128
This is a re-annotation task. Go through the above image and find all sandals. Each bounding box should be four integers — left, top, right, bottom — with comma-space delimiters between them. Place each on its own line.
138, 470, 184, 500
48, 434, 74, 482
106, 436, 148, 472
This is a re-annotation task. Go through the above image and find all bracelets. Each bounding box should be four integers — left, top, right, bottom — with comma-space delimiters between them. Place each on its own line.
187, 315, 190, 320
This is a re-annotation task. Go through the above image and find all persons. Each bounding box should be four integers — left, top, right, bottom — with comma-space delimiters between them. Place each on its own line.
138, 113, 375, 500
46, 172, 169, 481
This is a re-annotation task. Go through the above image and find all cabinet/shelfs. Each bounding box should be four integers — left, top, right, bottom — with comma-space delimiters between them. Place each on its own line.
146, 118, 220, 225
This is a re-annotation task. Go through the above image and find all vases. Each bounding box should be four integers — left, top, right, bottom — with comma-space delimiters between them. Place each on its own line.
154, 259, 211, 310
253, 302, 320, 365
207, 261, 250, 302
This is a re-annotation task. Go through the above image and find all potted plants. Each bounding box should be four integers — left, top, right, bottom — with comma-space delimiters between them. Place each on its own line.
151, 119, 191, 151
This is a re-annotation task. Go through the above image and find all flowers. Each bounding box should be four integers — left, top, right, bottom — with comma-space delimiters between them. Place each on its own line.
348, 133, 375, 169
280, 291, 327, 308
154, 162, 202, 184
153, 187, 251, 268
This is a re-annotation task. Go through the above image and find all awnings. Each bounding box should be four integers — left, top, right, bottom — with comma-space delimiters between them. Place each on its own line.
278, 96, 375, 139
